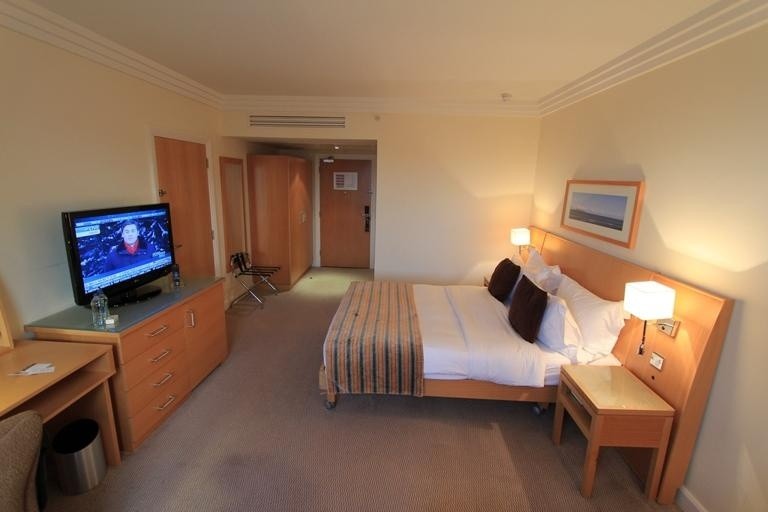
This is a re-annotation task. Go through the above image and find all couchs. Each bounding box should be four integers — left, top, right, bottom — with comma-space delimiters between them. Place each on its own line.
0, 411, 43, 512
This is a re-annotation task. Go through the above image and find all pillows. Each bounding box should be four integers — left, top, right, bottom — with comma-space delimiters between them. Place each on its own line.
508, 276, 547, 344
504, 255, 526, 304
525, 251, 560, 293
506, 281, 585, 363
488, 258, 521, 302
556, 273, 631, 362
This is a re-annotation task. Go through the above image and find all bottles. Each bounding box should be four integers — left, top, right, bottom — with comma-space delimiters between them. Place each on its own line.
90, 292, 107, 330
97, 290, 110, 319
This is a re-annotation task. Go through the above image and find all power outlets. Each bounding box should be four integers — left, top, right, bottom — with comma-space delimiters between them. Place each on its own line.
649, 352, 664, 371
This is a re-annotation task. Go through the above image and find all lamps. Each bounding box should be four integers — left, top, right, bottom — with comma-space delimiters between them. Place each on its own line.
623, 280, 680, 355
510, 227, 530, 257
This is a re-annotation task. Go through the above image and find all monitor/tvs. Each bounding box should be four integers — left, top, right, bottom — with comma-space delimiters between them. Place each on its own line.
60, 202, 176, 310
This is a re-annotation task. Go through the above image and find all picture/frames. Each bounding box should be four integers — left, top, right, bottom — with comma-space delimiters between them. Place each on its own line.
560, 178, 645, 250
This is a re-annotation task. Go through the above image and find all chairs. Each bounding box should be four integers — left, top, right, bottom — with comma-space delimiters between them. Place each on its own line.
230, 253, 281, 304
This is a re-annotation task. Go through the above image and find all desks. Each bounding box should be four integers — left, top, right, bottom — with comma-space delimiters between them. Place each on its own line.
24, 278, 228, 453
1, 301, 121, 469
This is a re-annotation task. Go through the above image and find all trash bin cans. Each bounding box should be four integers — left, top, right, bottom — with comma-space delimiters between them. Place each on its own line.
50, 417, 108, 495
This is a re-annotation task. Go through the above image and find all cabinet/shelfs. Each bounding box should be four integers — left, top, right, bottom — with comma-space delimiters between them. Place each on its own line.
246, 153, 312, 288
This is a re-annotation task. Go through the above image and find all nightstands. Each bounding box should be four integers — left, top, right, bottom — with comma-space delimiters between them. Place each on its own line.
554, 269, 735, 501
484, 226, 546, 286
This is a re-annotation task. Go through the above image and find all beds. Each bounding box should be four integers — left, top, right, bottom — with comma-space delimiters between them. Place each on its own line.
320, 233, 654, 411
